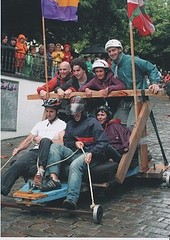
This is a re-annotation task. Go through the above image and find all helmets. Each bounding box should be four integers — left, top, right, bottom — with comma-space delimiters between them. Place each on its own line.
42, 98, 63, 107
105, 39, 123, 52
96, 105, 115, 121
66, 95, 87, 114
92, 59, 109, 71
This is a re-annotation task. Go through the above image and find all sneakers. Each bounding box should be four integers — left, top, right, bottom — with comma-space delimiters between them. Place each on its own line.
40, 177, 62, 191
63, 199, 76, 209
31, 174, 44, 189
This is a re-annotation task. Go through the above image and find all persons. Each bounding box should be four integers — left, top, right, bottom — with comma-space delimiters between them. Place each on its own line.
37, 61, 74, 112
80, 58, 127, 119
150, 63, 170, 95
55, 58, 95, 120
1, 31, 94, 85
0, 99, 73, 199
93, 105, 133, 163
104, 38, 159, 144
37, 96, 109, 212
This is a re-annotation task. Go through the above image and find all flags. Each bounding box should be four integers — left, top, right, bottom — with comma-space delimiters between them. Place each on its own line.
39, 0, 81, 22
125, 0, 159, 39
